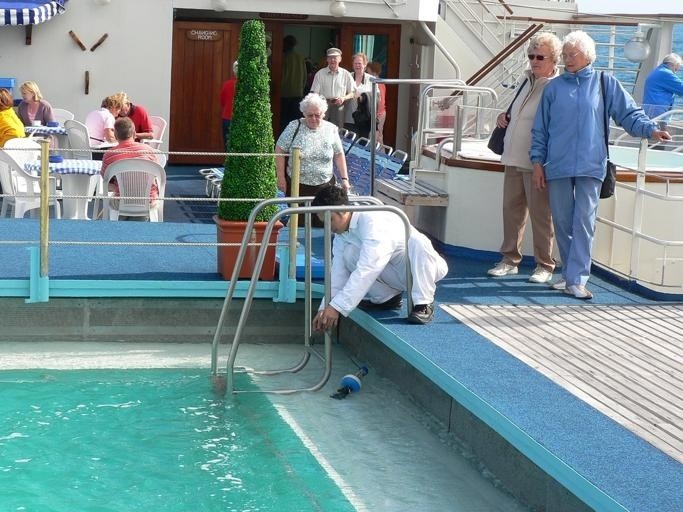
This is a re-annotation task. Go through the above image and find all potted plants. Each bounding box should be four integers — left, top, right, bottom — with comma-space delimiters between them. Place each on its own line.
212, 19, 283, 281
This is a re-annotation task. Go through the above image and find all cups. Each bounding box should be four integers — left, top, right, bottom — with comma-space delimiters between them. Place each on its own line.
32, 120, 41, 127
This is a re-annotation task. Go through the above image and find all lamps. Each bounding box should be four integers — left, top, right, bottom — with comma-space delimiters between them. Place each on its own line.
329, 0, 347, 18
624, 23, 661, 63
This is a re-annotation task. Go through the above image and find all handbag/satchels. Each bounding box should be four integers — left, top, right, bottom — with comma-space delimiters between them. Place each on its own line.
488, 116, 510, 155
598, 160, 615, 199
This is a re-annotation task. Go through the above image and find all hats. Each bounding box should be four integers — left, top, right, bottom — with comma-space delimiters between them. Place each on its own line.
326, 48, 342, 57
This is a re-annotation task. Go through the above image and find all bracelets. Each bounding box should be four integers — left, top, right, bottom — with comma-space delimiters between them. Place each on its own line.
341, 178, 350, 181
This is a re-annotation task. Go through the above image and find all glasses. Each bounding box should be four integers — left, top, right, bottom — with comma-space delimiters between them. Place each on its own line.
559, 49, 580, 59
527, 54, 551, 61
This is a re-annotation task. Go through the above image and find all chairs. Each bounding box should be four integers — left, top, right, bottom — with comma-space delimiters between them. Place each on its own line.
199, 127, 408, 225
0, 107, 166, 222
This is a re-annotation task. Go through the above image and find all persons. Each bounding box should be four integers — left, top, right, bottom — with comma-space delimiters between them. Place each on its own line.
275, 93, 353, 228
644, 54, 683, 131
0, 88, 26, 147
311, 185, 448, 333
119, 92, 153, 142
528, 28, 671, 298
487, 32, 563, 283
86, 95, 123, 160
19, 81, 56, 152
219, 60, 239, 151
97, 117, 158, 220
280, 35, 387, 155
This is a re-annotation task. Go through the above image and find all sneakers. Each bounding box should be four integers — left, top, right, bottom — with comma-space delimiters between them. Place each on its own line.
486, 261, 518, 276
529, 266, 552, 283
362, 295, 403, 309
408, 304, 434, 325
550, 278, 594, 299
